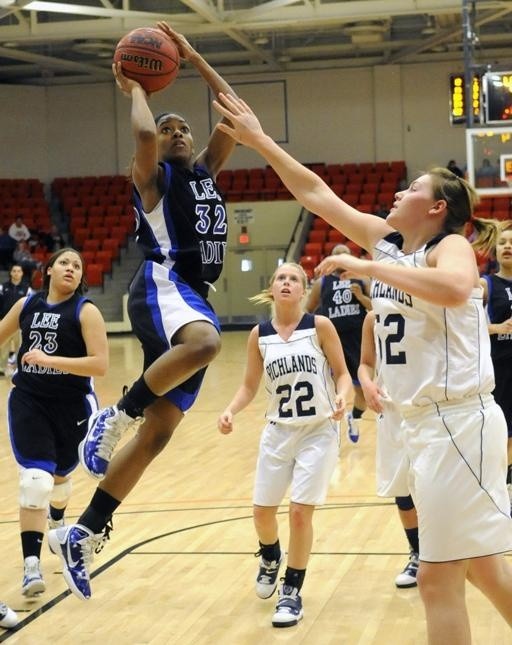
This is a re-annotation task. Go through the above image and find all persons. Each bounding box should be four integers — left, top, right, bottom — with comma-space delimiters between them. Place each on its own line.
1, 265, 37, 378
212, 90, 511, 645
1, 247, 109, 598
476, 160, 495, 177
45, 19, 244, 597
479, 218, 511, 492
357, 309, 419, 588
304, 244, 372, 442
447, 160, 464, 181
217, 261, 357, 629
1, 215, 65, 287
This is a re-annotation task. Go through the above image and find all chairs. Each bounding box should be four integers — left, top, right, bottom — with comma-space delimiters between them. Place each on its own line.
463, 177, 510, 236
217, 162, 406, 276
2, 177, 138, 292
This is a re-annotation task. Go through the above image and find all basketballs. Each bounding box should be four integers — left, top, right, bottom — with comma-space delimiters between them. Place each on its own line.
116, 27, 180, 91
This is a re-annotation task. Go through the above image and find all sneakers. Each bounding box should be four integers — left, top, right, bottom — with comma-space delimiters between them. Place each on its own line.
396, 551, 419, 588
0, 601, 17, 628
271, 583, 303, 627
46, 514, 65, 554
77, 404, 136, 480
22, 556, 46, 597
345, 411, 362, 443
255, 549, 284, 598
47, 524, 104, 601
